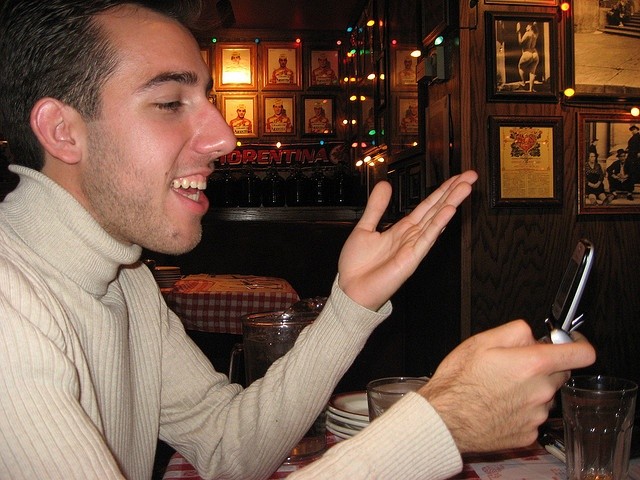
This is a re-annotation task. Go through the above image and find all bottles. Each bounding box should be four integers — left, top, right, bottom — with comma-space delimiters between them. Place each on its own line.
310, 159, 336, 207
337, 156, 356, 207
213, 162, 237, 208
235, 160, 260, 208
283, 161, 308, 206
261, 158, 284, 206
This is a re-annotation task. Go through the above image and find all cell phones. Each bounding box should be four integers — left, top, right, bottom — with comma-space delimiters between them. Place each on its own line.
538, 238, 595, 344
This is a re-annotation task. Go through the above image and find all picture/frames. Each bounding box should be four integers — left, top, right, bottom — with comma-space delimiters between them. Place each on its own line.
307, 47, 342, 91
483, 10, 559, 105
484, 0, 561, 7
212, 41, 257, 91
427, 47, 445, 86
420, 0, 449, 50
208, 94, 216, 105
577, 109, 639, 217
557, 0, 640, 100
300, 94, 337, 139
391, 42, 420, 93
258, 41, 303, 91
216, 92, 260, 141
200, 46, 211, 66
260, 92, 297, 137
426, 94, 450, 188
486, 115, 564, 215
396, 92, 420, 136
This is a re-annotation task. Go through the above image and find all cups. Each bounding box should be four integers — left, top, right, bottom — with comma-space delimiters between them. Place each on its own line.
560, 375, 638, 480
226, 311, 332, 465
366, 374, 428, 418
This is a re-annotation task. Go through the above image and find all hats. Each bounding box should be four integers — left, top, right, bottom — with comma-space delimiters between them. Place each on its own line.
615, 149, 627, 157
628, 126, 639, 132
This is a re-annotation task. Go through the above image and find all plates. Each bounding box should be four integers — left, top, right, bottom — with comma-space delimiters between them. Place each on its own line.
151, 265, 181, 287
325, 393, 368, 442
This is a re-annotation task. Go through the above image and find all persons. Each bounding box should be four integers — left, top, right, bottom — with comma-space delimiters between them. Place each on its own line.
402, 102, 419, 134
228, 105, 252, 133
585, 144, 608, 206
626, 125, 640, 186
607, 4, 625, 27
222, 51, 250, 83
270, 54, 294, 83
309, 102, 331, 133
616, 0, 635, 15
0, 1, 597, 480
267, 99, 292, 132
517, 21, 540, 92
606, 148, 634, 202
399, 56, 416, 82
311, 55, 338, 85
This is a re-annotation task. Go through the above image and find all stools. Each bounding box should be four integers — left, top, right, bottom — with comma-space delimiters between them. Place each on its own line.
229, 343, 246, 384
